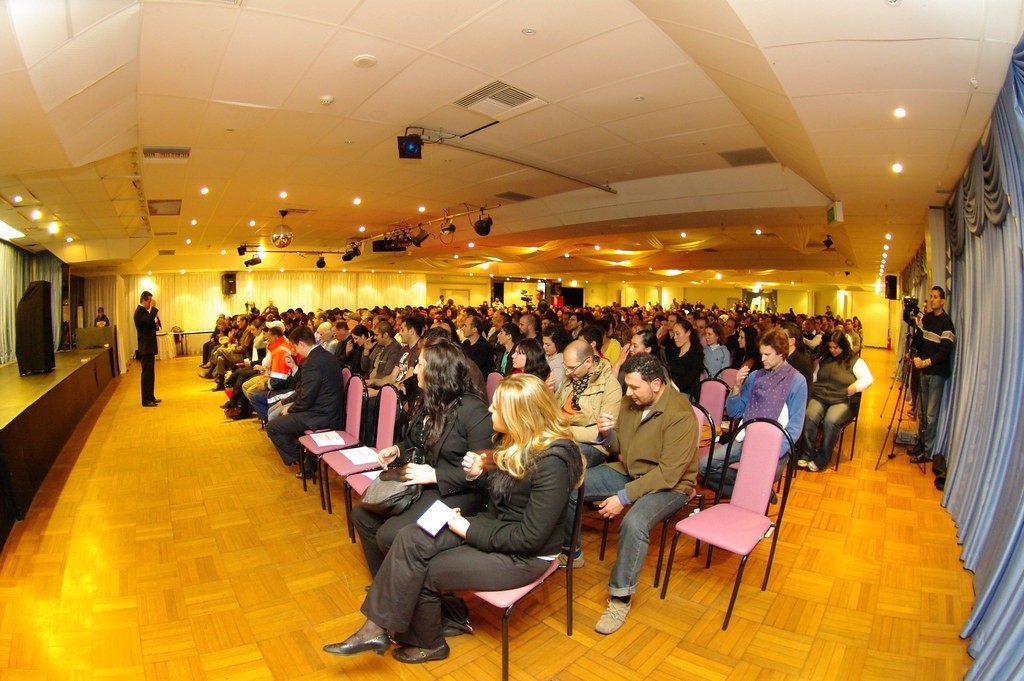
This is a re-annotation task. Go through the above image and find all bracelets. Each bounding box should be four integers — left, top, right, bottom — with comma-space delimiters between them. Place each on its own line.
922, 360, 926, 367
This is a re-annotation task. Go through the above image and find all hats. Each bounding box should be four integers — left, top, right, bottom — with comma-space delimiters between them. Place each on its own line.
266, 320, 286, 333
344, 312, 362, 322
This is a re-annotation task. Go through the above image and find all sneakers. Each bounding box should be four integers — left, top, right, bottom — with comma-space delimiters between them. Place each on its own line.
556, 552, 585, 568
595, 598, 633, 634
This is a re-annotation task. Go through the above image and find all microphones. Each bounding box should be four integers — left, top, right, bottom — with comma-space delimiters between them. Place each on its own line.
157, 316, 161, 328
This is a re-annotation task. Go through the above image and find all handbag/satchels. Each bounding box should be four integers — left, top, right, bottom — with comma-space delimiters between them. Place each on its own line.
360, 447, 426, 512
441, 593, 474, 637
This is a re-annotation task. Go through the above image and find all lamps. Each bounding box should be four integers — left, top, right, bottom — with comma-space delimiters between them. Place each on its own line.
237, 209, 494, 268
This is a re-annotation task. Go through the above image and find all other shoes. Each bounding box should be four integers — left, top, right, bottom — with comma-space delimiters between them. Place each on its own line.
798, 459, 808, 467
910, 454, 928, 463
296, 457, 315, 478
152, 398, 161, 403
907, 447, 922, 455
770, 489, 778, 505
808, 460, 818, 472
198, 360, 253, 420
143, 402, 158, 407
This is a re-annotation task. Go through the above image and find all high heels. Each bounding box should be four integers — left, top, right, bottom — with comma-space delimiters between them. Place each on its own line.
391, 640, 450, 663
323, 633, 391, 656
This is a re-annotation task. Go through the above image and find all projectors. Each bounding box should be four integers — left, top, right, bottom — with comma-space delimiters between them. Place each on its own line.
372, 240, 406, 252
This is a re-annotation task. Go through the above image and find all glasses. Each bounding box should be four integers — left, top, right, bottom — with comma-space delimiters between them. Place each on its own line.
560, 356, 591, 375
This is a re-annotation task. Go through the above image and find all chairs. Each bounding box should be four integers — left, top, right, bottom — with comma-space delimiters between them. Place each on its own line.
297, 366, 862, 681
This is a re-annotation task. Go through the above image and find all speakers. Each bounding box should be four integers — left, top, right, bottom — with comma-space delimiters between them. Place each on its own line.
885, 275, 897, 300
222, 274, 236, 294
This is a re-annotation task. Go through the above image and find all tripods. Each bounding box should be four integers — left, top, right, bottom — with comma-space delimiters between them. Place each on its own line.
875, 324, 926, 474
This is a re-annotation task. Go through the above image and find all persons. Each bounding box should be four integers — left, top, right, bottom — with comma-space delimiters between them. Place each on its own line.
134, 291, 162, 407
204, 296, 874, 664
906, 286, 955, 464
95, 307, 110, 327
